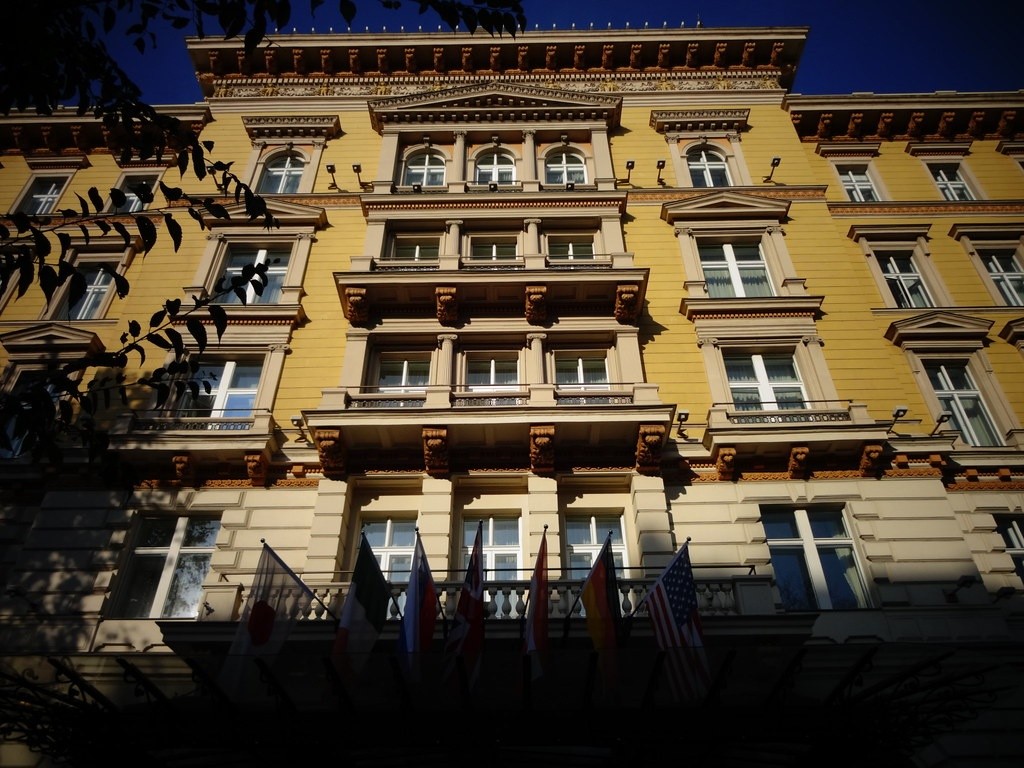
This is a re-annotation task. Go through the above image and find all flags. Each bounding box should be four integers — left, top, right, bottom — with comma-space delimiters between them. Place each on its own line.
215, 546, 313, 693
644, 547, 714, 701
317, 531, 623, 707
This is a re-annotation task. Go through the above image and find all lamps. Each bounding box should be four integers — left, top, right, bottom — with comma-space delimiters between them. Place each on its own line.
676, 410, 690, 438
352, 162, 368, 189
887, 405, 907, 434
762, 158, 781, 183
656, 159, 666, 185
951, 576, 1016, 605
621, 160, 635, 183
928, 411, 952, 436
488, 181, 498, 192
565, 181, 575, 191
325, 163, 339, 190
289, 415, 308, 442
411, 182, 423, 193
